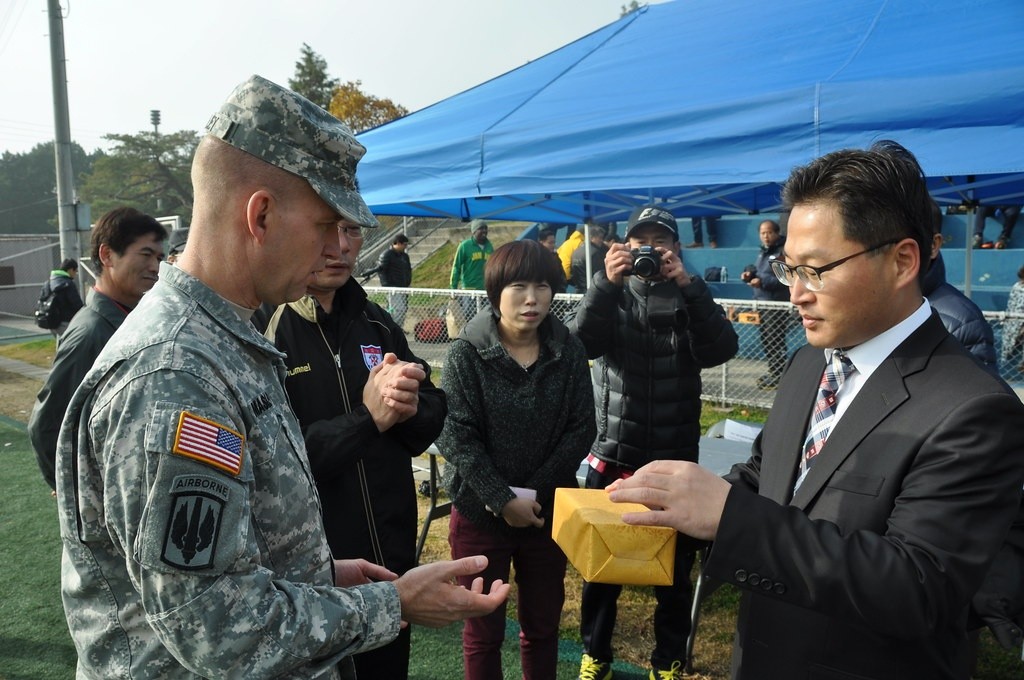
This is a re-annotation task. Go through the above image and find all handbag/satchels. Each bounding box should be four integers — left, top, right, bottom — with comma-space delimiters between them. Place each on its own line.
413, 319, 448, 343
446, 298, 468, 339
704, 266, 728, 282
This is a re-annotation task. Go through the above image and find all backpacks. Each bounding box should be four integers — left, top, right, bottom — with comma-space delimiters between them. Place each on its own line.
33, 279, 70, 330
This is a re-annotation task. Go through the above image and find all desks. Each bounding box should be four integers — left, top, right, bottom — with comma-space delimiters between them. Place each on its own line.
683, 418, 764, 674
416, 443, 451, 562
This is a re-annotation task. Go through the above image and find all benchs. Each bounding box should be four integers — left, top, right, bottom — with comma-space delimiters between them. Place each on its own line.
557, 206, 1024, 380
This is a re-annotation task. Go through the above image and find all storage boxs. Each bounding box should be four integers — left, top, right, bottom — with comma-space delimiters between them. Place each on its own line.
551, 488, 677, 587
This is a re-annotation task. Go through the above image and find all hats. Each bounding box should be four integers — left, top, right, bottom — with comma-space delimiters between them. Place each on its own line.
168, 227, 189, 254
470, 218, 487, 234
203, 73, 381, 228
623, 205, 679, 246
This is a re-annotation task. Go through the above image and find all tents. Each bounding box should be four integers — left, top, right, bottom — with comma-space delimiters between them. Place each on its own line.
347, 0, 1024, 311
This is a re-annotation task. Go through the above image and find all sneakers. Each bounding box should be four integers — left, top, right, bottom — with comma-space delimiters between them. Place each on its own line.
574, 654, 613, 680
648, 660, 681, 680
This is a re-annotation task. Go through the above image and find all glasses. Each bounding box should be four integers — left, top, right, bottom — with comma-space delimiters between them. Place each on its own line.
770, 237, 906, 291
74, 269, 79, 274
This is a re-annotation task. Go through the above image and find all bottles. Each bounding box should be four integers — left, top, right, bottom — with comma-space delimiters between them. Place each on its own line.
720, 266, 726, 283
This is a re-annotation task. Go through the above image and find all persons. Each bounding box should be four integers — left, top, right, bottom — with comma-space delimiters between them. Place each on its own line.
56, 74, 510, 680
27, 206, 169, 497
741, 219, 788, 391
947, 204, 1022, 249
158, 228, 191, 282
534, 222, 624, 323
449, 218, 495, 322
376, 234, 412, 335
1000, 267, 1024, 383
609, 141, 1024, 679
571, 205, 741, 679
920, 203, 995, 371
255, 217, 450, 679
433, 238, 598, 680
33, 258, 83, 338
685, 215, 722, 248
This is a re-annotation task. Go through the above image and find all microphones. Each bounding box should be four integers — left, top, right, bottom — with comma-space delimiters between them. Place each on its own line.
360, 263, 386, 277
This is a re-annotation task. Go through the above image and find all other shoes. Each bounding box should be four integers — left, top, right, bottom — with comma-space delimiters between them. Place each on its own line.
995, 238, 1008, 249
971, 235, 983, 248
756, 374, 783, 390
684, 240, 703, 248
710, 240, 717, 248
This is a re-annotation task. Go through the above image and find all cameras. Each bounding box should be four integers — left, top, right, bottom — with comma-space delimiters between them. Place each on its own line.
621, 245, 666, 276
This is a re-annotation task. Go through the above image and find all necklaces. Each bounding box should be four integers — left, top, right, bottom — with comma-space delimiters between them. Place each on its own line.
500, 335, 536, 370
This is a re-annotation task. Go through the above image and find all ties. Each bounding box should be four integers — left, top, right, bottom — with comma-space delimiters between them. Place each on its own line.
792, 350, 857, 498
